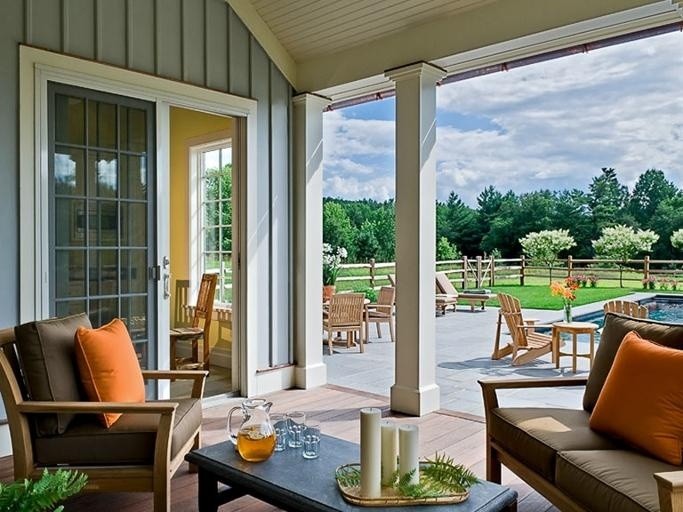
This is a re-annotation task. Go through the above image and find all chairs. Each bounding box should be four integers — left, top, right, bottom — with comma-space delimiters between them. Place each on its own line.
0, 313, 210, 512
603, 300, 649, 319
492, 292, 565, 366
170, 273, 218, 382
323, 272, 497, 356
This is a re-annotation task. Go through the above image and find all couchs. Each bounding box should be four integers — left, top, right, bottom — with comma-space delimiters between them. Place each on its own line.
477, 311, 683, 512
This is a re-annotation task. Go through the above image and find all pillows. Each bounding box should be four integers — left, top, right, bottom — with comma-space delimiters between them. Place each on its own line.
75, 318, 146, 429
589, 330, 683, 466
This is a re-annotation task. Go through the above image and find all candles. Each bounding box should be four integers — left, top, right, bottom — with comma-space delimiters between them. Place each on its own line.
361, 407, 419, 497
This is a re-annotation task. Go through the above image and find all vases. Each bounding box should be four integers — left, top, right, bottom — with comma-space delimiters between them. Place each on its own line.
644, 284, 655, 289
577, 280, 596, 288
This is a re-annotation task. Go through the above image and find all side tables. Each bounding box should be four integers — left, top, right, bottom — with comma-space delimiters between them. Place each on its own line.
552, 322, 599, 373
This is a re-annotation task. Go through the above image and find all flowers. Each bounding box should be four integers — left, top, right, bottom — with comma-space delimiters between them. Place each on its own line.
564, 274, 656, 285
550, 277, 579, 324
323, 243, 348, 285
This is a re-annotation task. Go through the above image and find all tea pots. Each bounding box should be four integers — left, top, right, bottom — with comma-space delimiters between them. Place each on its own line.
227, 398, 276, 462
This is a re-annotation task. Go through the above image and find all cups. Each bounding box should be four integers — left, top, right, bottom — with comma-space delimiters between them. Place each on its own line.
285, 411, 305, 448
269, 413, 287, 452
300, 422, 320, 459
